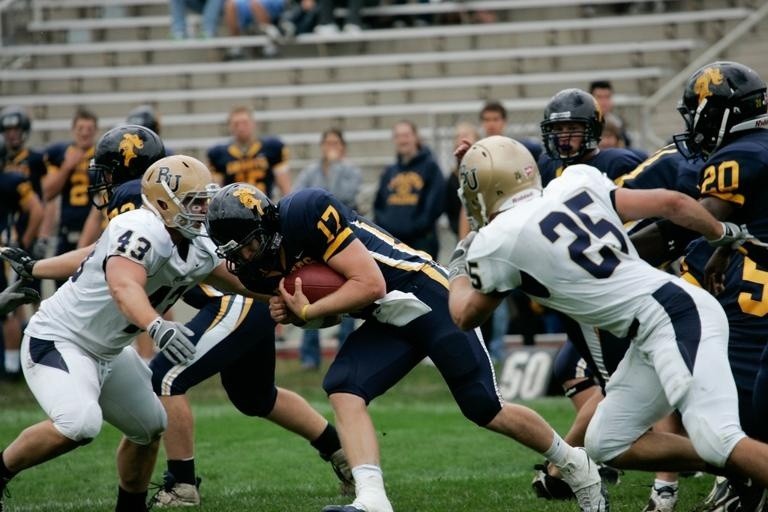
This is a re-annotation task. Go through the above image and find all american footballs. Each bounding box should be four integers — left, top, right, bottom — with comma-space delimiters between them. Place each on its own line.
285, 264, 346, 303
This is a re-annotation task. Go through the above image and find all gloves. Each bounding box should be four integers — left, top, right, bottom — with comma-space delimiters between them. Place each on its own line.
0, 245, 35, 284
0, 277, 42, 316
706, 221, 754, 254
446, 229, 480, 285
146, 317, 197, 366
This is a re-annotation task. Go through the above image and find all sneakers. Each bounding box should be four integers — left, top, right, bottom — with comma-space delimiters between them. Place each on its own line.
296, 362, 319, 375
319, 446, 354, 494
166, 22, 364, 60
692, 476, 768, 512
639, 479, 681, 512
321, 494, 395, 512
529, 448, 623, 511
144, 470, 202, 510
0, 366, 23, 383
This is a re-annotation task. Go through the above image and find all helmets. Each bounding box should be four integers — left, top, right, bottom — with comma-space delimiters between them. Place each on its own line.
456, 133, 543, 231
139, 153, 215, 243
85, 124, 166, 212
204, 181, 282, 275
538, 88, 605, 164
0, 109, 33, 151
672, 60, 768, 165
121, 104, 161, 135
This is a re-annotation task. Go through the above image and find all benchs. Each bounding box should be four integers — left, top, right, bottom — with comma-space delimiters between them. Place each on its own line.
0, 0, 768, 268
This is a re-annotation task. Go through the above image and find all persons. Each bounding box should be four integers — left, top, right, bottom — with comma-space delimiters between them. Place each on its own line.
2, 120, 366, 512
1, 153, 277, 512
627, 55, 766, 509
676, 216, 767, 512
437, 137, 766, 512
529, 125, 712, 512
203, 178, 610, 511
534, 90, 648, 485
